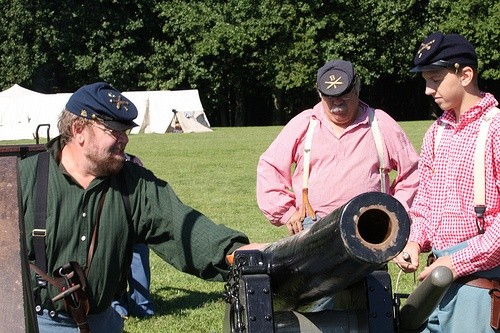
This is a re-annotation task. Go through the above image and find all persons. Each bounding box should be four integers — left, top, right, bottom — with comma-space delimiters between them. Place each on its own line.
393, 32, 500, 333
256, 59, 420, 312
110, 152, 155, 319
18, 82, 267, 333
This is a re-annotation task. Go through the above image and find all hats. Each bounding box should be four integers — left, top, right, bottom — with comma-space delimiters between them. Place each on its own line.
314, 60, 356, 97
410, 32, 480, 73
65, 82, 140, 132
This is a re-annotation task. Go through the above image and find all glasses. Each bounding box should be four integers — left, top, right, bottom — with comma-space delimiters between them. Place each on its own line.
86, 121, 132, 140
321, 84, 358, 101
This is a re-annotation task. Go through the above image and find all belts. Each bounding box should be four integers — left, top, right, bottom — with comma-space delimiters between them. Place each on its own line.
427, 256, 500, 332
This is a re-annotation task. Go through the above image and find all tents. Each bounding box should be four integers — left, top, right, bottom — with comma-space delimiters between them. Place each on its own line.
0, 84, 213, 140
